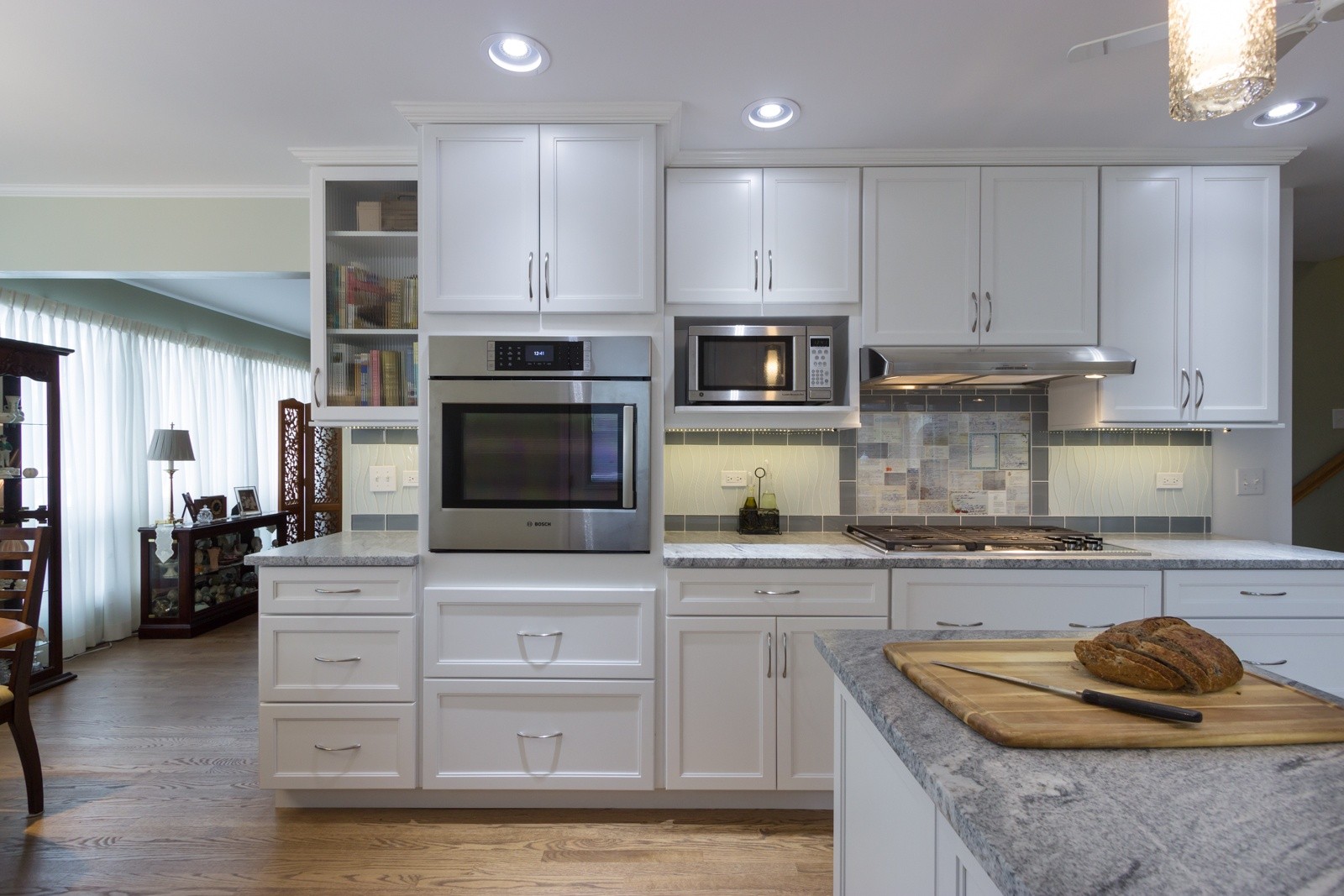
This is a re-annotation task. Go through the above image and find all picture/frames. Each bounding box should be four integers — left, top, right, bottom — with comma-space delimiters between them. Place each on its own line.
233, 485, 262, 517
182, 492, 198, 525
137, 510, 293, 640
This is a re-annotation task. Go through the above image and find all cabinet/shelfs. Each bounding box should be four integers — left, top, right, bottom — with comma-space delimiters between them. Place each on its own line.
1163, 570, 1344, 699
666, 168, 862, 306
308, 165, 419, 429
665, 616, 890, 791
665, 567, 890, 617
891, 566, 1162, 632
1099, 162, 1281, 429
420, 586, 657, 793
419, 122, 658, 316
256, 565, 419, 790
1, 334, 77, 707
861, 165, 1098, 348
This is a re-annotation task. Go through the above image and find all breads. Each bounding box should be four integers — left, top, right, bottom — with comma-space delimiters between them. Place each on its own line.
1075, 617, 1244, 694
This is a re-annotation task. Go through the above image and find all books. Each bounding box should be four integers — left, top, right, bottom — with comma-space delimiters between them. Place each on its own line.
325, 261, 418, 330
369, 348, 383, 406
360, 350, 370, 407
380, 349, 403, 406
402, 341, 419, 406
330, 337, 363, 407
354, 352, 363, 407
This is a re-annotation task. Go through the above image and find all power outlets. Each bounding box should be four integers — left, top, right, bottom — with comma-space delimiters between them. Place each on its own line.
403, 470, 418, 487
1156, 472, 1183, 490
721, 470, 748, 488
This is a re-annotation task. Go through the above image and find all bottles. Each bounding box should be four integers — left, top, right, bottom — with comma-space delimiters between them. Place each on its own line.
744, 484, 757, 530
761, 472, 777, 529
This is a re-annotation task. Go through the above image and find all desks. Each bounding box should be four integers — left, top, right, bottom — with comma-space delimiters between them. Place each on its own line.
0, 616, 34, 656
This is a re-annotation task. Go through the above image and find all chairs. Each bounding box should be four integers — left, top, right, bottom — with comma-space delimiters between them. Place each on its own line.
0, 525, 55, 817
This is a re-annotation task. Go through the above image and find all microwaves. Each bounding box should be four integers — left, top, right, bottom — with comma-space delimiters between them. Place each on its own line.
687, 325, 833, 403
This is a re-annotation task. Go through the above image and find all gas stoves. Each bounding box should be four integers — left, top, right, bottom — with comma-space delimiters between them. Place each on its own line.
845, 525, 1103, 553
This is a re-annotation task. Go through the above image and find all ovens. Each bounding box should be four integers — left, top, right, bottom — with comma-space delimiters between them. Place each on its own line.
427, 333, 651, 552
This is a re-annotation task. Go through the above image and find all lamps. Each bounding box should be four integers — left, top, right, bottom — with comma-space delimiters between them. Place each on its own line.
1168, 0, 1278, 124
146, 422, 196, 529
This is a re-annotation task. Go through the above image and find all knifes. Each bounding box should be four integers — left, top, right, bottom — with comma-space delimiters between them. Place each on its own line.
929, 659, 1203, 722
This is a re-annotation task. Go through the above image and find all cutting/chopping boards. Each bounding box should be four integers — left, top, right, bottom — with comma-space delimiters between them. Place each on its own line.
883, 640, 1344, 748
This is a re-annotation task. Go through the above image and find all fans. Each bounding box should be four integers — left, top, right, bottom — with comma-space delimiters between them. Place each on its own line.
1066, 0, 1344, 64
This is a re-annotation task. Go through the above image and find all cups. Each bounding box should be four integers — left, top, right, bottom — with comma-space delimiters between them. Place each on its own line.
4, 395, 24, 422
197, 504, 213, 523
0, 413, 16, 423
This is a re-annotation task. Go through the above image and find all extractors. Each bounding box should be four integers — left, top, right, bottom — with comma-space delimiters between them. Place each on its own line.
860, 344, 1136, 388
